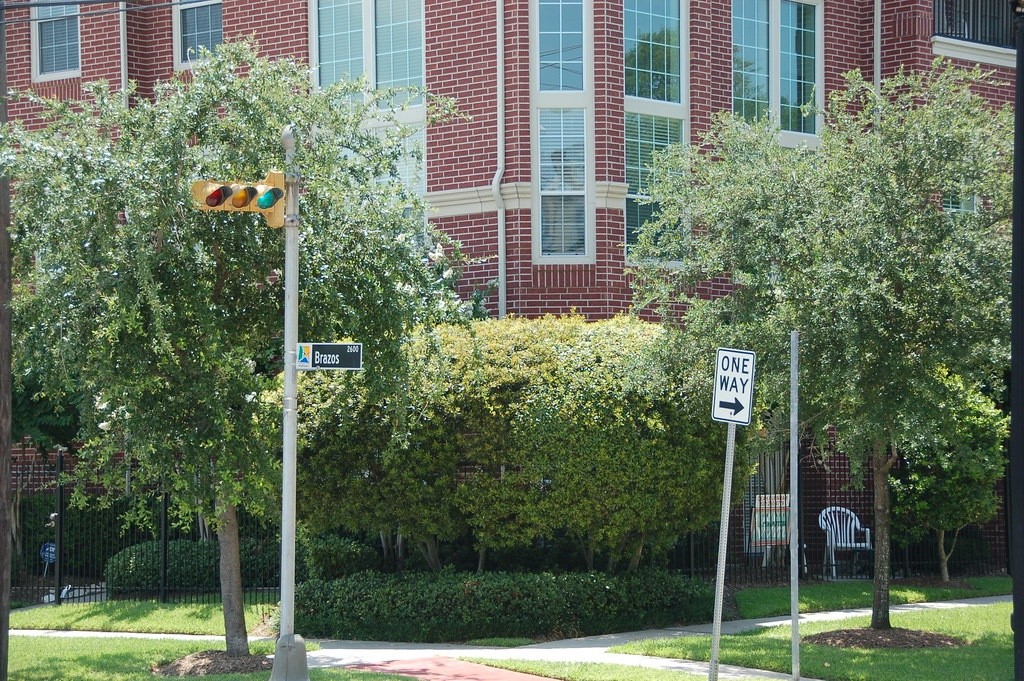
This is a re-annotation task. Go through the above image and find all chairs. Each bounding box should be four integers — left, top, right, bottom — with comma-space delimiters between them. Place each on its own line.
818, 507, 872, 578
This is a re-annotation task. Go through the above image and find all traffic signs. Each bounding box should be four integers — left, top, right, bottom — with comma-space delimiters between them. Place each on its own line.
713, 347, 756, 427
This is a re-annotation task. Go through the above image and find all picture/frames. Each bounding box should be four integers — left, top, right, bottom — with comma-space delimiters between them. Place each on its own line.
750, 507, 790, 546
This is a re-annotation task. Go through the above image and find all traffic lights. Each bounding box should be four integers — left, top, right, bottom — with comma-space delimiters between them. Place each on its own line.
191, 175, 284, 213
